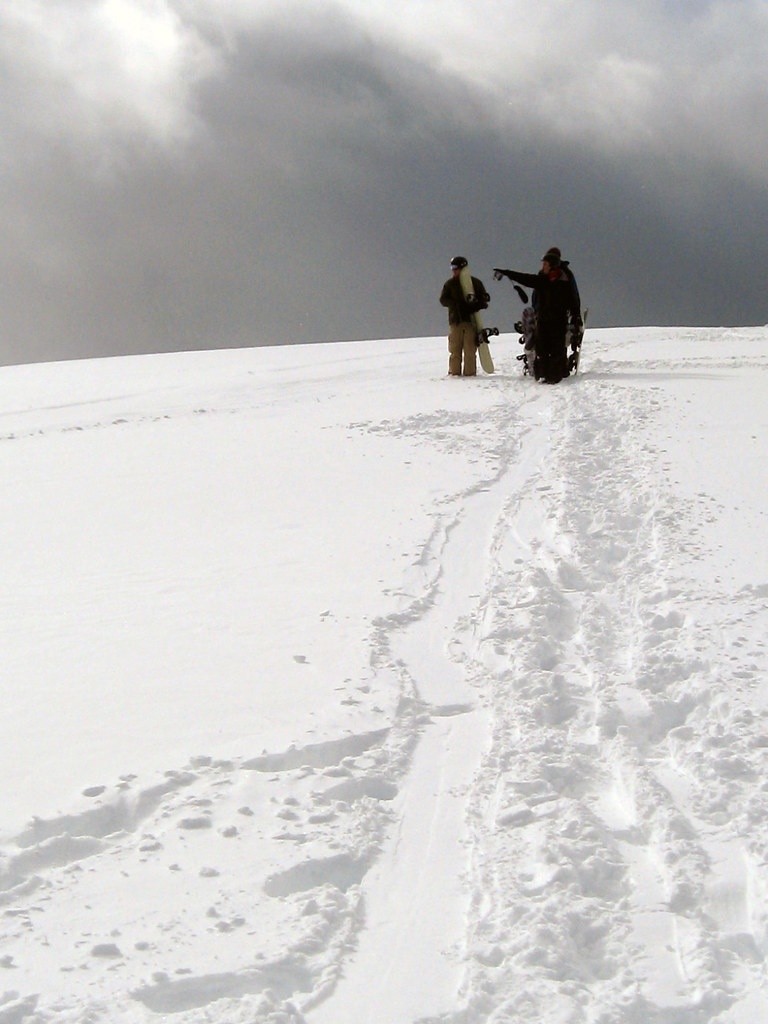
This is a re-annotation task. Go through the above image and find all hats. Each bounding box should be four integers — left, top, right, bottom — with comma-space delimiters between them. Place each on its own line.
542, 248, 561, 271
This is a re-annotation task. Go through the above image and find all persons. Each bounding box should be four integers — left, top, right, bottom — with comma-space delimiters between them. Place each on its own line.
439, 256, 491, 376
493, 247, 583, 384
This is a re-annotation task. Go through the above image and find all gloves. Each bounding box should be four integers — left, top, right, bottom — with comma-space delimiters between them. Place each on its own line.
493, 268, 508, 280
570, 314, 583, 333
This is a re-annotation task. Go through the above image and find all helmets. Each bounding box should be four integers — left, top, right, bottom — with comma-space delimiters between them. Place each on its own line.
450, 256, 467, 270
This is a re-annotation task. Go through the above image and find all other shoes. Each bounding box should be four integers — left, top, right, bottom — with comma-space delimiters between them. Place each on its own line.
541, 379, 558, 385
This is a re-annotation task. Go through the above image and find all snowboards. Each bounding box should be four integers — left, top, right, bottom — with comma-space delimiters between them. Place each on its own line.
575, 306, 590, 375
521, 306, 539, 377
459, 266, 494, 374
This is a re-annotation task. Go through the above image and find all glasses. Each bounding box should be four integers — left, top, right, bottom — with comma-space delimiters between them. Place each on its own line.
451, 265, 457, 270
